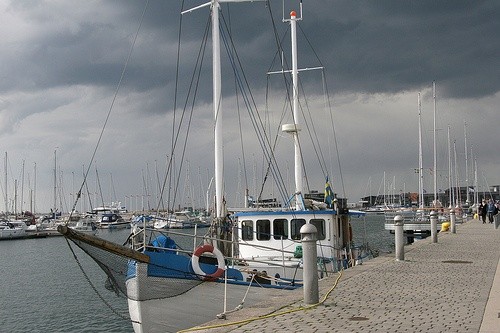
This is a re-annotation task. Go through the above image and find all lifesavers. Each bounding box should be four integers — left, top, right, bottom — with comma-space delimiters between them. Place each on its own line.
192, 245, 225, 282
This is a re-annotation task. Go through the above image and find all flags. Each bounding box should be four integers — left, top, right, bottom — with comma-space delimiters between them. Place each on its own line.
324, 177, 337, 208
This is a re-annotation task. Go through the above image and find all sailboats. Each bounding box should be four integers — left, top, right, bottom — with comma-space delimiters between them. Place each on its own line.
0, 149, 211, 241
57, 0, 378, 333
346, 79, 482, 238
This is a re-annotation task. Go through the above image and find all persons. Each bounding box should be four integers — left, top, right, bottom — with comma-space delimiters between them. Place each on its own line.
257, 270, 271, 285
486, 198, 495, 224
478, 199, 488, 224
245, 269, 259, 284
485, 199, 500, 218
